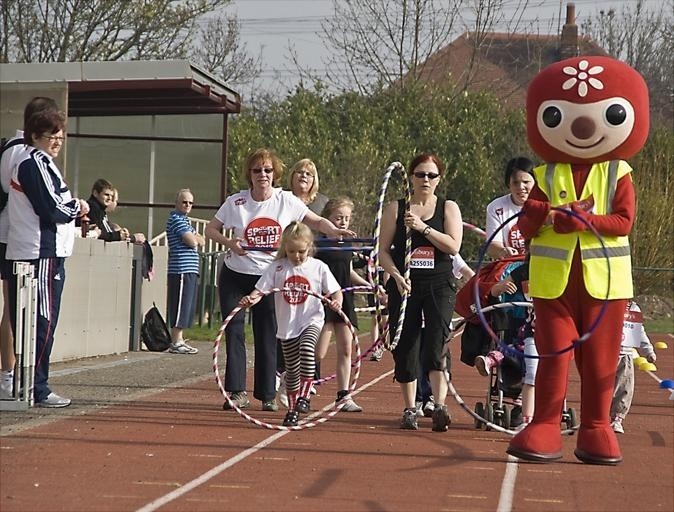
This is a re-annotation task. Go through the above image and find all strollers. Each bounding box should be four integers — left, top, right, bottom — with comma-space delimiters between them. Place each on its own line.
455, 255, 576, 430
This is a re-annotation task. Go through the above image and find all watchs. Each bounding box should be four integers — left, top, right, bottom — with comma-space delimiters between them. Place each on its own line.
422, 226, 432, 237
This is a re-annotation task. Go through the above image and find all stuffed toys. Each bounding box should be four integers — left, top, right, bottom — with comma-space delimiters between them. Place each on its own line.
505, 55, 651, 467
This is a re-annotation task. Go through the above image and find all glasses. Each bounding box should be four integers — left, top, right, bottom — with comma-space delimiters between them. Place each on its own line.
180, 200, 195, 205
251, 167, 274, 174
412, 172, 441, 180
43, 135, 66, 143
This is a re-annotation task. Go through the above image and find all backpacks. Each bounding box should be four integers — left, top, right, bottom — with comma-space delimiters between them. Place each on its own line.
141, 302, 172, 353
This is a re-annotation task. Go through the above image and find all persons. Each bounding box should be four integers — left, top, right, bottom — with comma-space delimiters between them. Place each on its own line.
311, 197, 362, 414
102, 186, 147, 247
240, 222, 342, 427
0, 96, 61, 404
486, 157, 545, 431
279, 158, 330, 226
5, 110, 91, 408
609, 299, 657, 433
375, 154, 462, 432
167, 189, 204, 353
492, 238, 539, 440
78, 179, 129, 245
403, 246, 476, 416
205, 150, 356, 410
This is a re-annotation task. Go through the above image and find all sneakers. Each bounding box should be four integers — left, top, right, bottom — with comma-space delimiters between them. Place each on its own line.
431, 406, 451, 432
613, 419, 625, 433
335, 397, 364, 413
263, 398, 278, 411
295, 397, 311, 410
0, 369, 15, 401
413, 401, 437, 417
36, 392, 72, 408
370, 348, 383, 362
224, 391, 251, 410
170, 343, 198, 354
400, 409, 420, 430
284, 410, 298, 426
476, 355, 491, 376
516, 421, 529, 430
